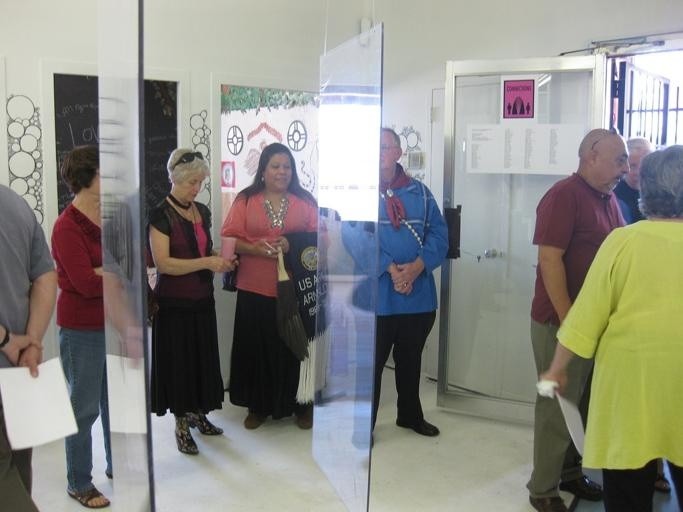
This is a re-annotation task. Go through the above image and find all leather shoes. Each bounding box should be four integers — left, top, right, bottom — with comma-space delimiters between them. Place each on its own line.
396, 419, 439, 437
298, 405, 313, 429
529, 496, 568, 512
560, 475, 603, 501
245, 412, 267, 429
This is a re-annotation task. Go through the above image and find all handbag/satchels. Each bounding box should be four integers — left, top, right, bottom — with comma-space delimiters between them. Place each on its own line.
222, 271, 237, 292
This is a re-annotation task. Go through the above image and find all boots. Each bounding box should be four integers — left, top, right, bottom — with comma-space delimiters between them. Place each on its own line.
185, 409, 223, 435
175, 416, 198, 454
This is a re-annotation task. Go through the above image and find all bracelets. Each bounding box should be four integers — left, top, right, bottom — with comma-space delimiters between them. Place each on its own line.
0, 324, 10, 349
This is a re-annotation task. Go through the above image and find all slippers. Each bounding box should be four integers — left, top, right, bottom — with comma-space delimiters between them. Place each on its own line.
67, 488, 110, 508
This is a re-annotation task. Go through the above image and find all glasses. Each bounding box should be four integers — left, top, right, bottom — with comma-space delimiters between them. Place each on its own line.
173, 152, 204, 169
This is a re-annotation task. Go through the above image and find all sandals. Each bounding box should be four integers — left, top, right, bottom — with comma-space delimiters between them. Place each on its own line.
654, 473, 672, 494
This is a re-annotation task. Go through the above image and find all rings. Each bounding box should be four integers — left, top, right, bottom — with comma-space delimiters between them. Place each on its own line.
267, 250, 271, 256
403, 282, 407, 287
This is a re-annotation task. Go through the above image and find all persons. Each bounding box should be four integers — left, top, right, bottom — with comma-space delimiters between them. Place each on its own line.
0, 169, 77, 511
611, 137, 674, 497
141, 148, 235, 459
53, 145, 143, 508
102, 186, 140, 370
338, 128, 456, 451
525, 128, 632, 511
535, 142, 683, 511
218, 142, 333, 430
